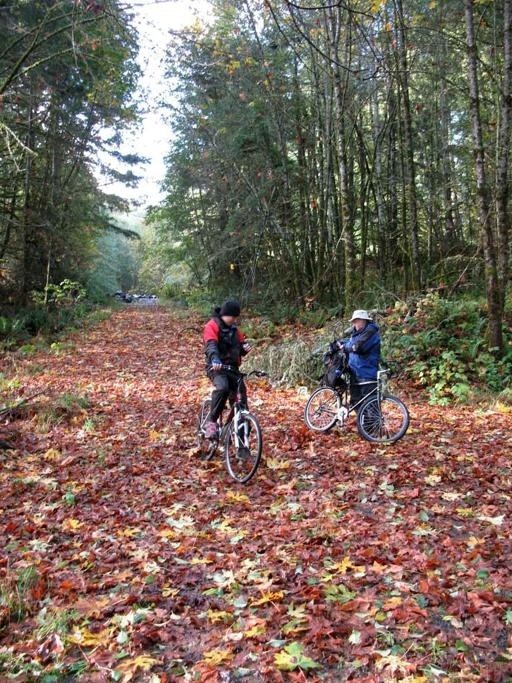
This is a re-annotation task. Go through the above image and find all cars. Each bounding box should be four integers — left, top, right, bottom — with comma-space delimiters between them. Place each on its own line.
114, 290, 160, 303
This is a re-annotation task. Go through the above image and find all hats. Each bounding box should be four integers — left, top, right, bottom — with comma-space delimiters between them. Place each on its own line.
219, 300, 241, 317
348, 308, 374, 322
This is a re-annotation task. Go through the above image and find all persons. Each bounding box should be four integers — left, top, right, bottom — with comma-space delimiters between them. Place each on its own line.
201, 300, 252, 461
333, 308, 383, 432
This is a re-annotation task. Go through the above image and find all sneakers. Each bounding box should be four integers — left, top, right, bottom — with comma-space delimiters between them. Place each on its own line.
204, 421, 221, 441
236, 447, 257, 463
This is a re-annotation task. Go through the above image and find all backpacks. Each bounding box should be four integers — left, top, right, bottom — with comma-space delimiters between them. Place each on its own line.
321, 341, 352, 389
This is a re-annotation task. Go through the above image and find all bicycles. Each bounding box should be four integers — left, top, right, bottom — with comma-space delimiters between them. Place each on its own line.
197, 364, 267, 485
304, 359, 410, 445
